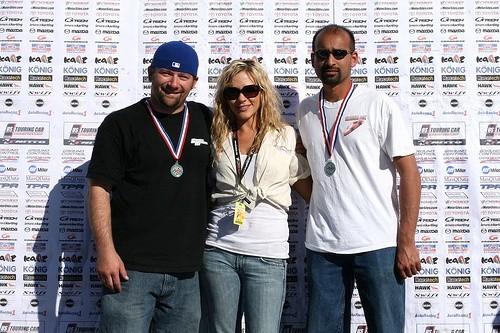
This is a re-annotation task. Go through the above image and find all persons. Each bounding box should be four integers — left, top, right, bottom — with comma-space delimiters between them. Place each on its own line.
85, 40, 306, 333
202, 58, 313, 333
296, 22, 422, 333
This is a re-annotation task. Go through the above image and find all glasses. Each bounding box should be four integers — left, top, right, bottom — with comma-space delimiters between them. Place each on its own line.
223, 84, 259, 99
314, 49, 354, 60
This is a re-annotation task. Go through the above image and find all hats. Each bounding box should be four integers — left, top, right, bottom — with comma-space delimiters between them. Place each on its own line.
150, 41, 199, 77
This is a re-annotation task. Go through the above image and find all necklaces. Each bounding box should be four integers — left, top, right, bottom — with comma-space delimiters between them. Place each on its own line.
230, 123, 261, 226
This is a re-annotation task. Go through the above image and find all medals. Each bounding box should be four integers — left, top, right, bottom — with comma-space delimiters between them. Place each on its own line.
170, 161, 184, 177
324, 162, 336, 176
